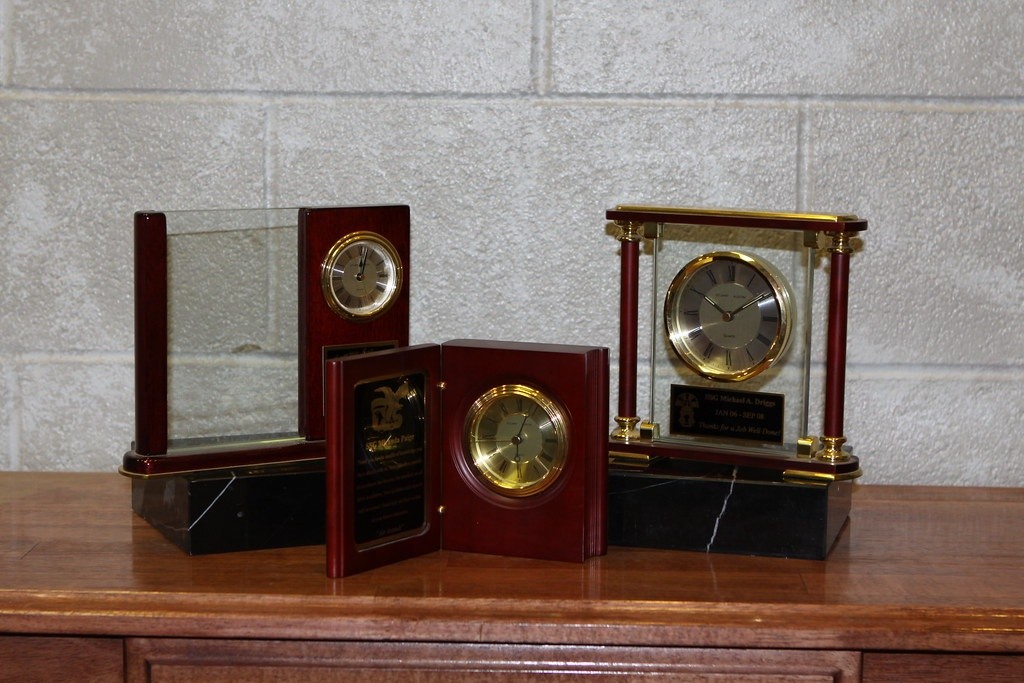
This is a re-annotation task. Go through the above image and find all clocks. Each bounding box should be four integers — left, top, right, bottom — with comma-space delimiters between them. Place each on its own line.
326, 342, 610, 579
118, 205, 410, 558
602, 208, 869, 563
